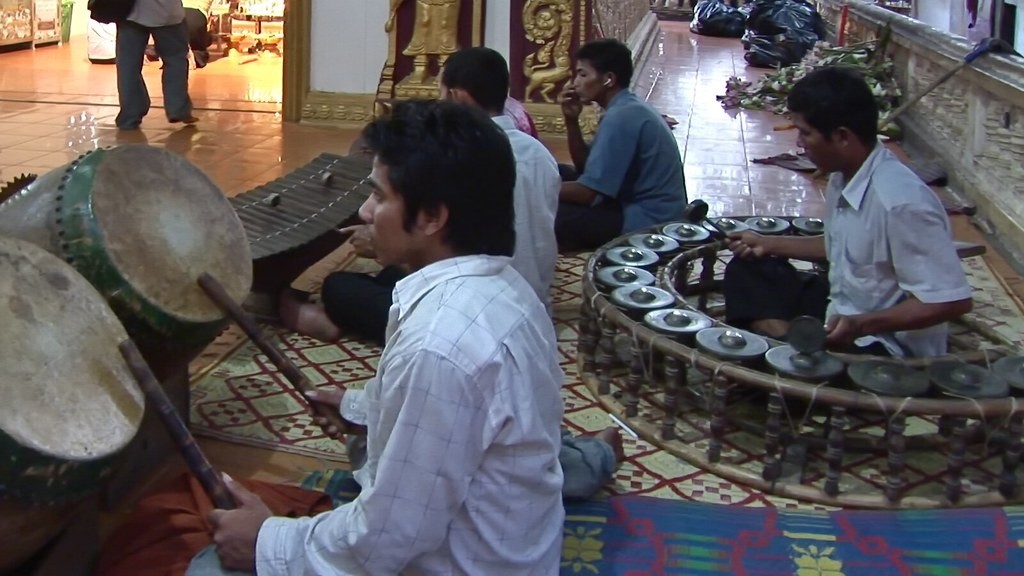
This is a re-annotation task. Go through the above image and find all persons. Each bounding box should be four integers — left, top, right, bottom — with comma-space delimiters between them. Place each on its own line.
276, 39, 688, 345
115, 0, 199, 129
722, 67, 974, 419
87, 0, 212, 70
188, 97, 624, 576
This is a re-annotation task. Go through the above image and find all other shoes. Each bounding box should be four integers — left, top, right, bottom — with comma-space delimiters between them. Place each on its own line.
169, 116, 201, 131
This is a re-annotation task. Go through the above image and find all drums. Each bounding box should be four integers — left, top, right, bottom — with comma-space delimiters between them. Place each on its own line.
0, 233, 147, 508
0, 142, 252, 384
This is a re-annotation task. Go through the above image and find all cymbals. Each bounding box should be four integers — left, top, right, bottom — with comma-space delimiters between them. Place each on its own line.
745, 217, 790, 233
696, 327, 769, 359
847, 361, 930, 396
644, 308, 712, 333
993, 356, 1024, 389
596, 265, 656, 288
702, 218, 750, 233
792, 217, 824, 232
765, 345, 844, 379
611, 286, 675, 312
930, 363, 1009, 399
628, 234, 679, 252
662, 223, 710, 242
606, 247, 660, 267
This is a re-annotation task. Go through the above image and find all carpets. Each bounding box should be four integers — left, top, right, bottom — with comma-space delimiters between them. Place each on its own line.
277, 460, 1024, 576
191, 253, 1024, 506
